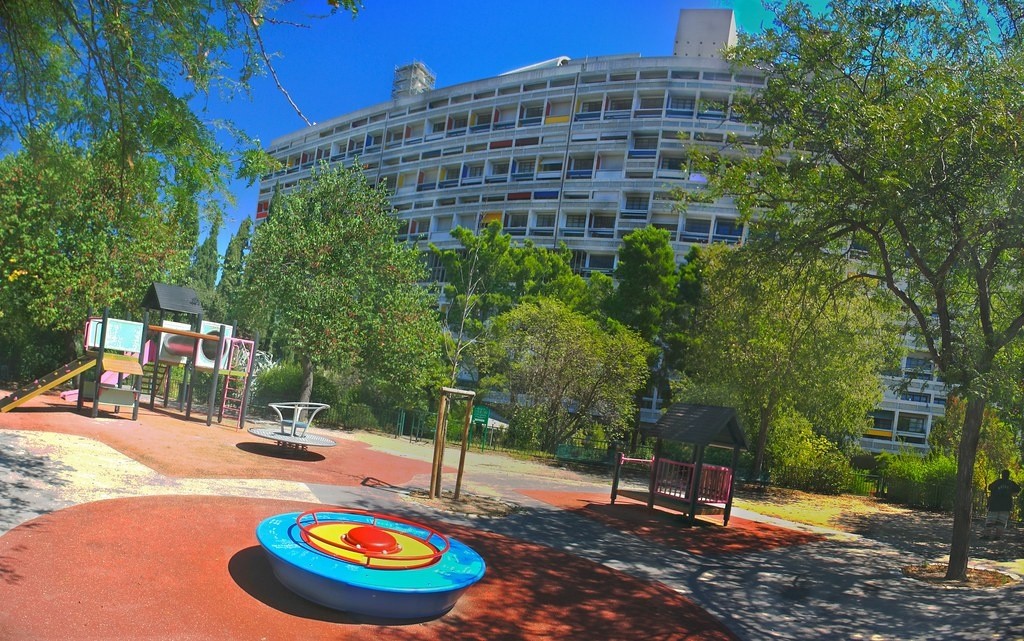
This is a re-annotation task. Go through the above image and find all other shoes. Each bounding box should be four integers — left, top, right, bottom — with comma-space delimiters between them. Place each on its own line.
995, 536, 1000, 540
979, 535, 989, 540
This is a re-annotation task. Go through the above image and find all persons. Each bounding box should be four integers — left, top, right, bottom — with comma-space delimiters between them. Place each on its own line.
980, 470, 1021, 540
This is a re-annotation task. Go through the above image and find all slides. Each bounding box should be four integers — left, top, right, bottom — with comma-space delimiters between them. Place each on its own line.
0, 355, 97, 413
